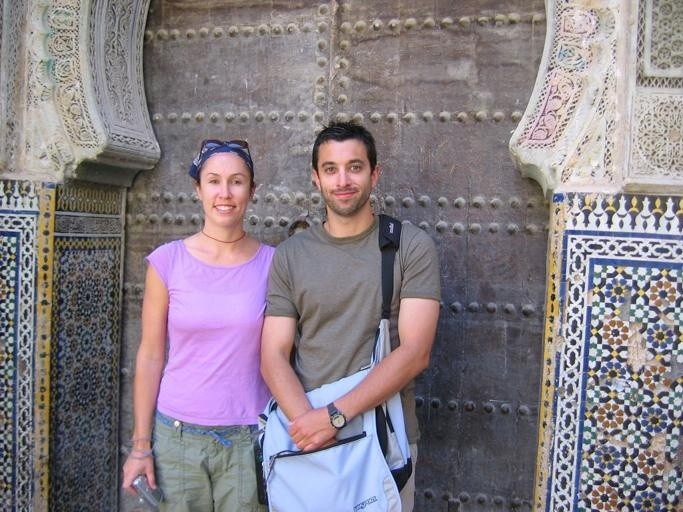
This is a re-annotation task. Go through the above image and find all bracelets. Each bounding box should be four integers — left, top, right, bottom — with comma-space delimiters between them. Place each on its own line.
122, 437, 155, 460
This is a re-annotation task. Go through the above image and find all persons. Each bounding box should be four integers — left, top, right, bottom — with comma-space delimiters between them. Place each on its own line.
256, 118, 441, 512
122, 138, 277, 512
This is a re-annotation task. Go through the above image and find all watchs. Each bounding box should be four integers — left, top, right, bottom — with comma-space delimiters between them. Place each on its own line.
326, 402, 347, 430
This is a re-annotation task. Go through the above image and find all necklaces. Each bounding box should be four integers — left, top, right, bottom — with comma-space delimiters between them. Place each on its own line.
201, 228, 245, 243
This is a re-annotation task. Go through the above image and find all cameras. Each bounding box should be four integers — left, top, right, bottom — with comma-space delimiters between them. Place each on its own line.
131, 474, 163, 509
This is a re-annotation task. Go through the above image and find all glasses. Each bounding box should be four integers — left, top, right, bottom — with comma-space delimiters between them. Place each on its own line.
198, 139, 252, 165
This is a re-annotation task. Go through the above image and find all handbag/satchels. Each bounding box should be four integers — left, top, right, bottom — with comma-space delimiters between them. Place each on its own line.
257, 214, 413, 512
